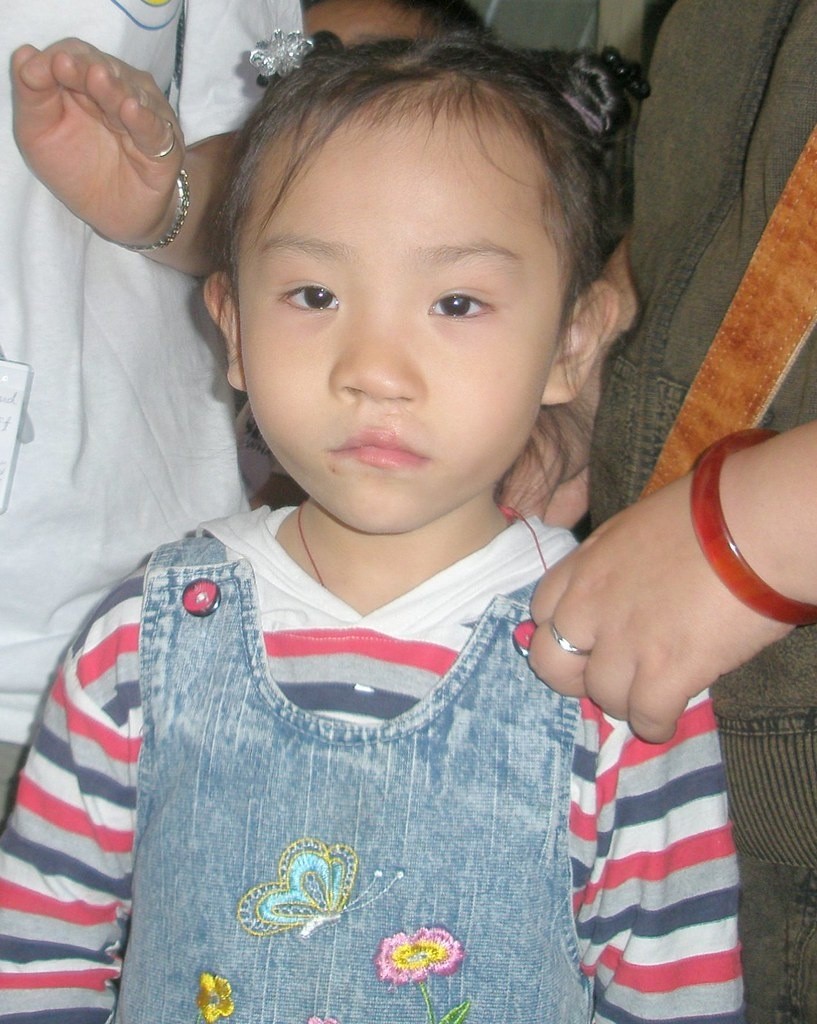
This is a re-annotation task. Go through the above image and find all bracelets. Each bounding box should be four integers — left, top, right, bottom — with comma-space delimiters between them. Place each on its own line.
689, 427, 817, 626
105, 168, 191, 253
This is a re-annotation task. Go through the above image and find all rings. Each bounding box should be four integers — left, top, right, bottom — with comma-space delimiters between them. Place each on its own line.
142, 121, 178, 161
547, 618, 592, 660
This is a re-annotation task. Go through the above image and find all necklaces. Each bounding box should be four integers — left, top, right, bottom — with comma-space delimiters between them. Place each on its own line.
295, 503, 548, 613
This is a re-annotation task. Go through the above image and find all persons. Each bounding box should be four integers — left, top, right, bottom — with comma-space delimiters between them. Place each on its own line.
0, 0, 817, 1024
